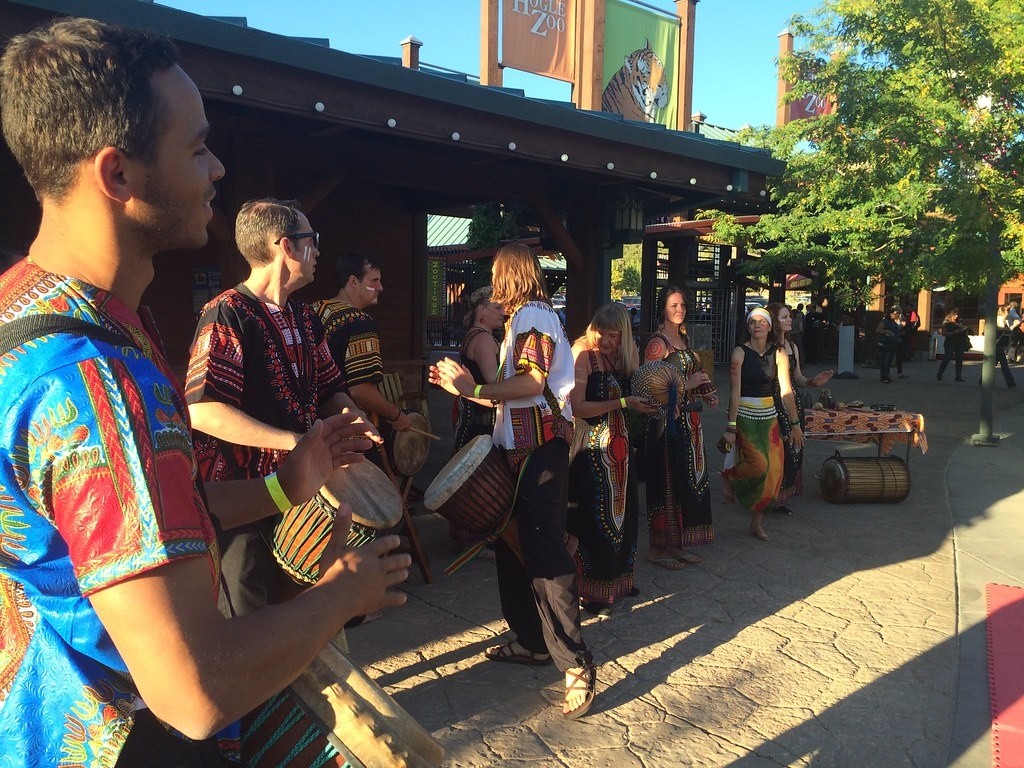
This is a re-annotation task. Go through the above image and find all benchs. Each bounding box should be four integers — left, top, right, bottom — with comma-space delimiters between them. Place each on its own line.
931, 334, 985, 357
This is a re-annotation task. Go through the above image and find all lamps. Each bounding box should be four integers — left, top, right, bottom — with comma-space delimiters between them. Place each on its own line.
609, 193, 646, 249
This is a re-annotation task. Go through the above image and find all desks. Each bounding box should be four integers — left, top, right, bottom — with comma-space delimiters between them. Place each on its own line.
804, 405, 927, 463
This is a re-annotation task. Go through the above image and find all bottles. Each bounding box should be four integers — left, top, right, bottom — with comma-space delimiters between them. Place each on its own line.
799, 392, 813, 409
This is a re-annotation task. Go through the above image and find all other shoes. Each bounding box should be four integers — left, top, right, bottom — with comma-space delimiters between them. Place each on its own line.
937, 374, 941, 381
776, 506, 794, 515
956, 378, 965, 382
578, 597, 611, 617
898, 375, 908, 379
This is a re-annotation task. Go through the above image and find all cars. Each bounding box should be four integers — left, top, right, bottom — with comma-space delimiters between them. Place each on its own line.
551, 293, 811, 330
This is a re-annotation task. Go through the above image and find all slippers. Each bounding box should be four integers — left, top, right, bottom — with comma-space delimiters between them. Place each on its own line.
677, 551, 702, 563
646, 554, 683, 570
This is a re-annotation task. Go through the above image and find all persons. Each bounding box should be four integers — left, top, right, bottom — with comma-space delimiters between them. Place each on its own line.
428, 244, 597, 719
627, 304, 825, 369
937, 306, 969, 383
448, 286, 719, 618
876, 304, 917, 384
0, 17, 410, 768
559, 307, 565, 326
184, 197, 410, 628
765, 303, 834, 516
978, 301, 1024, 389
723, 306, 807, 541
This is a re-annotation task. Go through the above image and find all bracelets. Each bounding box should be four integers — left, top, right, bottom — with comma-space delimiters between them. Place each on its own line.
264, 472, 293, 512
807, 378, 817, 388
620, 397, 627, 408
728, 421, 736, 426
791, 420, 800, 425
726, 427, 738, 434
392, 411, 401, 421
473, 385, 482, 398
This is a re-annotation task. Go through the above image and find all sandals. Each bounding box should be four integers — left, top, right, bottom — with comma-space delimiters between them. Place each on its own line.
483, 644, 554, 665
562, 664, 598, 719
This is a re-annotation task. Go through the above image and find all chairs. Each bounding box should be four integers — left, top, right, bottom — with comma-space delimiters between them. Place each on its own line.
374, 368, 434, 425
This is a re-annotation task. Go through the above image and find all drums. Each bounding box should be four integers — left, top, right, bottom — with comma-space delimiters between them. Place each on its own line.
269, 452, 403, 584
378, 408, 432, 476
423, 434, 579, 569
240, 639, 445, 768
820, 454, 911, 504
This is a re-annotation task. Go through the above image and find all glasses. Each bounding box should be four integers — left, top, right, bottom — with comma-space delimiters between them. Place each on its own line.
274, 232, 322, 248
